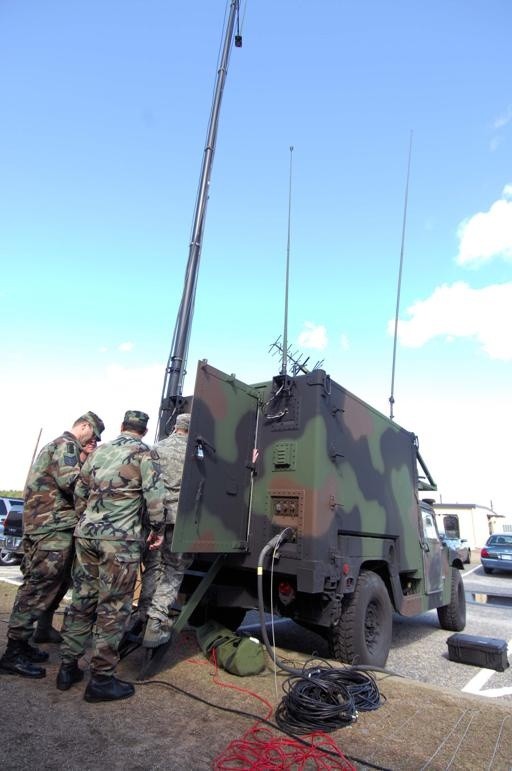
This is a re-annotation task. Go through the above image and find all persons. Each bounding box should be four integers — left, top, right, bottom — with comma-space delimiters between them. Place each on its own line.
34, 434, 102, 646
143, 414, 193, 647
0, 413, 104, 680
57, 409, 167, 703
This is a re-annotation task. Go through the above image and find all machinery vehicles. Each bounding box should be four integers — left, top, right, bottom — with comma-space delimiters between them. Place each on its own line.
166, 365, 470, 673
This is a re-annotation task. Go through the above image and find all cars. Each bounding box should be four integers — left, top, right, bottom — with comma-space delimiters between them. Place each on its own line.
480, 533, 512, 575
436, 532, 472, 565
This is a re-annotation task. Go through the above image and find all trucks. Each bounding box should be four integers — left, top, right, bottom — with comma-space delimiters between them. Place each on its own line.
2, 504, 26, 556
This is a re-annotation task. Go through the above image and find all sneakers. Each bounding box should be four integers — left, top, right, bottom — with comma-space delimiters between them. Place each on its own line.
7, 633, 51, 665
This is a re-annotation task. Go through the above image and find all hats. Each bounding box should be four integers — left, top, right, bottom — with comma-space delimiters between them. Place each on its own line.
121, 410, 150, 428
174, 413, 191, 432
82, 411, 106, 443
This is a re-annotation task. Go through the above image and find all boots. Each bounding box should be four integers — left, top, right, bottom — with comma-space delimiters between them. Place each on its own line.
141, 615, 172, 654
125, 607, 149, 647
31, 614, 66, 644
1, 641, 48, 681
83, 673, 136, 704
54, 659, 86, 693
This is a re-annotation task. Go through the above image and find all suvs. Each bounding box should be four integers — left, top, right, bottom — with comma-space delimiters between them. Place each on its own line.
0, 495, 26, 567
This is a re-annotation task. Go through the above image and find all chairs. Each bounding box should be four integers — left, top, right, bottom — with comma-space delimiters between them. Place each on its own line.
498, 538, 505, 542
